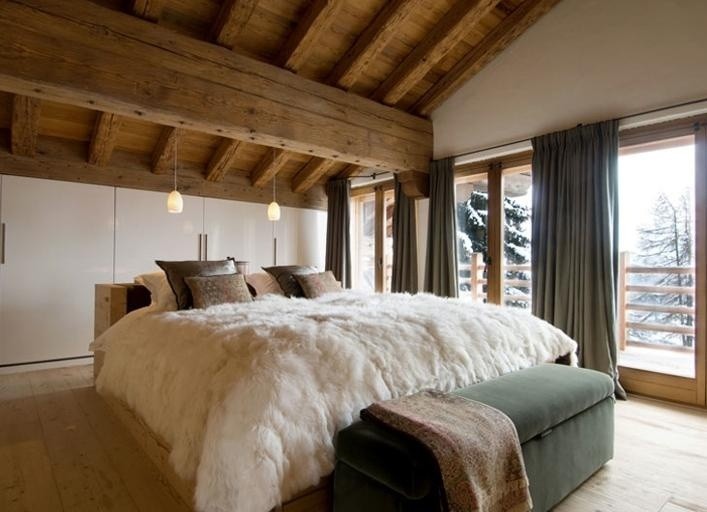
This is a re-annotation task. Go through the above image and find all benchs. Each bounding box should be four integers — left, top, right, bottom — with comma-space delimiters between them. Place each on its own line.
338, 360, 616, 506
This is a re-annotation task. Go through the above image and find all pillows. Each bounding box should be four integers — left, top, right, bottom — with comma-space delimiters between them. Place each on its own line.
138, 257, 345, 310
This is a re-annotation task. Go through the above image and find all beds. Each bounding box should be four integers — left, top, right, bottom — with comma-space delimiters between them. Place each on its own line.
92, 253, 583, 508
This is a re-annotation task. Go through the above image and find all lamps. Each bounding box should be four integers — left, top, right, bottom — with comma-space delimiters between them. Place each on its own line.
166, 129, 185, 214
267, 148, 283, 220
